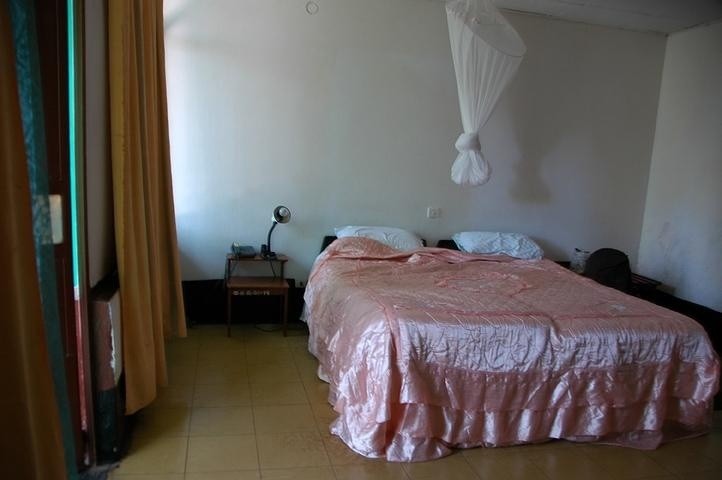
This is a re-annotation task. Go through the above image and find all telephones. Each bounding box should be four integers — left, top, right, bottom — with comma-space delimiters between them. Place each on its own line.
232, 242, 256, 258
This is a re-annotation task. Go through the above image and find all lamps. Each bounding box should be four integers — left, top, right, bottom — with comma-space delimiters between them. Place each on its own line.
260, 205, 291, 261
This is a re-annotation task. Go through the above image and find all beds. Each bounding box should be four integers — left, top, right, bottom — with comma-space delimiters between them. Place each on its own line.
304, 239, 719, 461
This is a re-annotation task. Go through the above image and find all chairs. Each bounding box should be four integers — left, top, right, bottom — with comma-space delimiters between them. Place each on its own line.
583, 247, 631, 291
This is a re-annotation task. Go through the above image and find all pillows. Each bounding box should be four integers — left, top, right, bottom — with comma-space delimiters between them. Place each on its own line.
450, 231, 545, 258
333, 224, 423, 251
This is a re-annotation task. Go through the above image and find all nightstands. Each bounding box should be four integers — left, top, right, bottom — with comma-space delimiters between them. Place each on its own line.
225, 252, 288, 338
